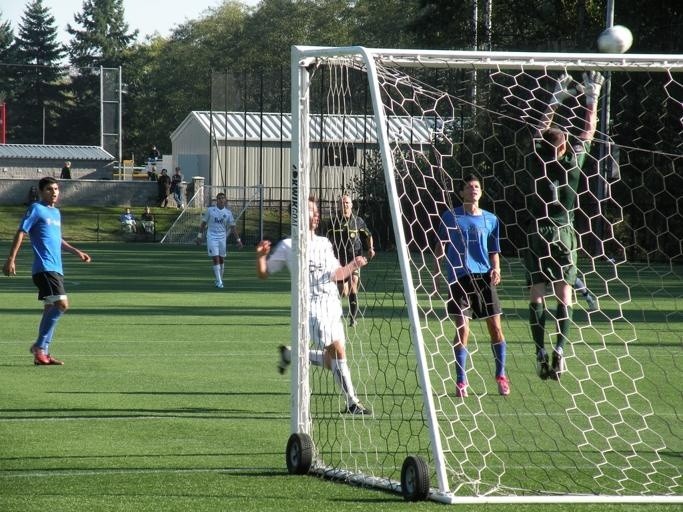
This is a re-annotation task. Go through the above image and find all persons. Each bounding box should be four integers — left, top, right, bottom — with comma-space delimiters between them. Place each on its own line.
325, 194, 376, 326
512, 70, 604, 380
256, 197, 372, 415
147, 166, 158, 181
141, 206, 157, 232
59, 162, 70, 178
4, 176, 90, 365
168, 168, 185, 209
121, 208, 137, 232
197, 193, 244, 289
434, 174, 510, 396
149, 145, 159, 161
158, 169, 171, 209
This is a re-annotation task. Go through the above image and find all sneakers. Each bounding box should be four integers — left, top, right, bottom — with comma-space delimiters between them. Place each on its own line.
536, 348, 550, 380
34, 354, 65, 367
213, 279, 224, 290
275, 344, 291, 376
584, 292, 597, 311
495, 375, 511, 396
455, 381, 468, 397
30, 343, 50, 365
344, 401, 372, 415
349, 319, 357, 327
550, 346, 564, 381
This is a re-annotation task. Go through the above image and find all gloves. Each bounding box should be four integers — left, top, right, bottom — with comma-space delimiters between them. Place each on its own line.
574, 69, 606, 107
548, 72, 578, 108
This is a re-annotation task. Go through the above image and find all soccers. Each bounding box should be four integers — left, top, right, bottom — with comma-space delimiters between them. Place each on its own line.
600, 26, 634, 52
